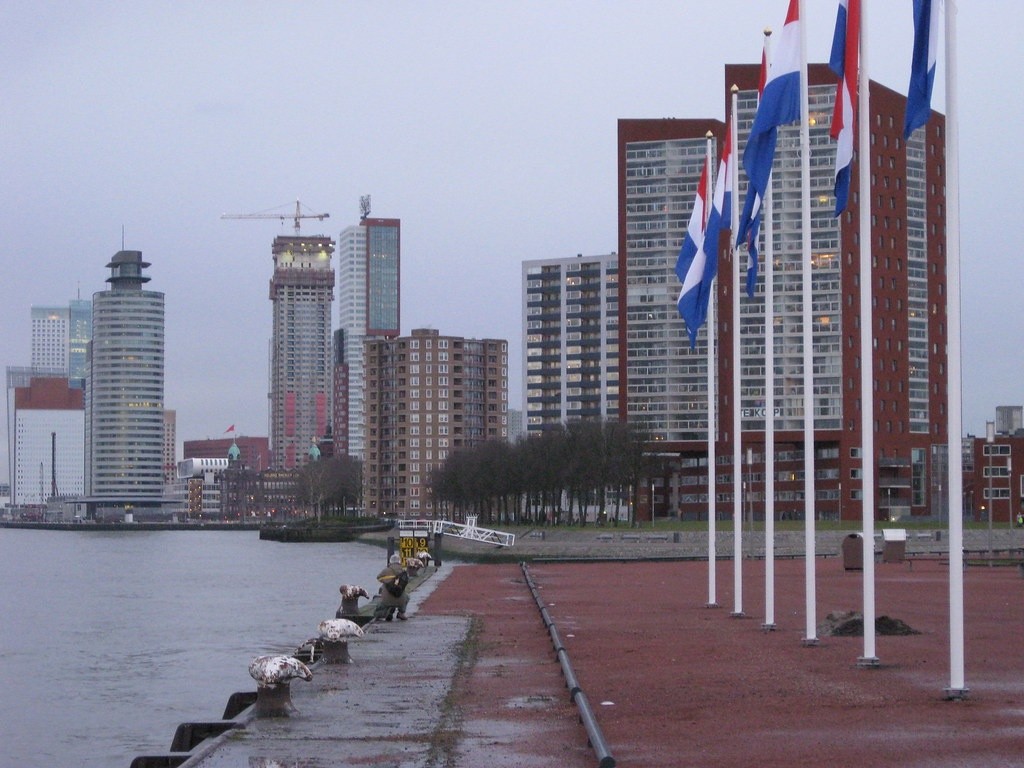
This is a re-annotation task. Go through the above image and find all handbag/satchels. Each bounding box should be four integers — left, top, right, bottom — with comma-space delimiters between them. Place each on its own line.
387, 571, 408, 597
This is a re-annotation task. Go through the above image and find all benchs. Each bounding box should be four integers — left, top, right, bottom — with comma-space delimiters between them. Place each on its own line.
899, 556, 949, 572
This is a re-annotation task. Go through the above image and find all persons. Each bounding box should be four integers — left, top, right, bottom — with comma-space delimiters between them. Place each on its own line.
1016, 511, 1022, 528
377, 554, 410, 621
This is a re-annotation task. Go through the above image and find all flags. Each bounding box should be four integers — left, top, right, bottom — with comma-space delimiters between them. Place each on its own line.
746, 0, 801, 194
900, 0, 940, 139
735, 50, 768, 298
224, 425, 235, 433
704, 115, 733, 286
674, 158, 708, 351
828, 1, 862, 218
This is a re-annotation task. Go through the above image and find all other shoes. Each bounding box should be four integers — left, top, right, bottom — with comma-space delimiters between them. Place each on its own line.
397, 612, 408, 620
385, 607, 395, 621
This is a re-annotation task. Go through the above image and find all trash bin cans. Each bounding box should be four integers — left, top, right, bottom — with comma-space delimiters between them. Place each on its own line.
842, 533, 863, 571
883, 528, 907, 561
